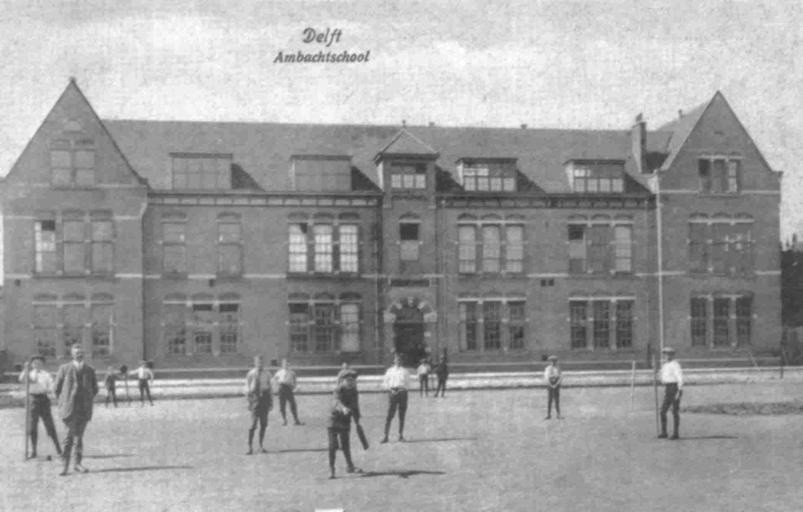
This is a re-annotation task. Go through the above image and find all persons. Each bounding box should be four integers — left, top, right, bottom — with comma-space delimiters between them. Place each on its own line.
103, 366, 126, 408
325, 354, 450, 480
53, 343, 99, 475
19, 355, 62, 459
543, 354, 565, 419
130, 358, 155, 407
272, 359, 306, 426
244, 352, 275, 455
657, 343, 685, 440
